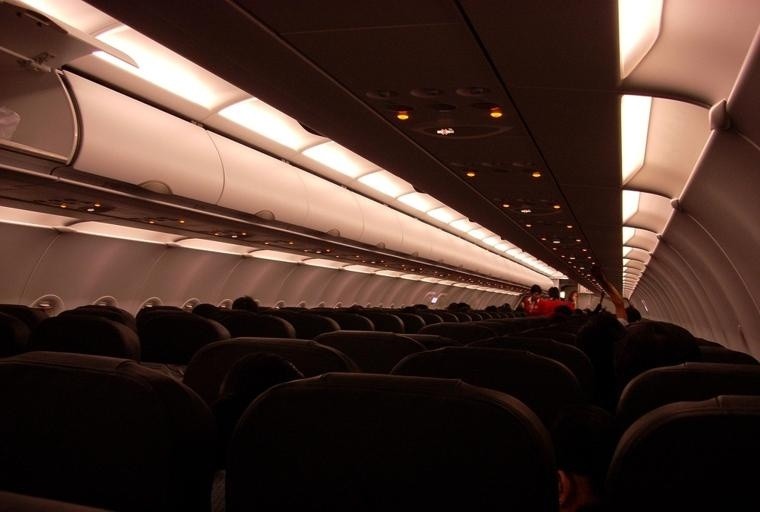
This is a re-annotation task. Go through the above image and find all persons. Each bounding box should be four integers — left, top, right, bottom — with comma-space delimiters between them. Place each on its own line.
552, 304, 643, 336
614, 317, 703, 400
533, 286, 575, 318
543, 401, 627, 512
402, 301, 520, 317
516, 284, 544, 317
230, 296, 261, 316
565, 289, 579, 309
207, 352, 305, 511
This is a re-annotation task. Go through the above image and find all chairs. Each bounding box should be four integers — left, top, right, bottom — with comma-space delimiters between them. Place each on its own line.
0, 301, 760, 511
